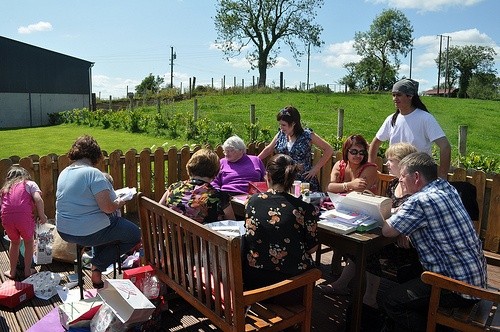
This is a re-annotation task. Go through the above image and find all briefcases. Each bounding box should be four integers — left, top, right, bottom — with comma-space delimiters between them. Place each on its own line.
346, 190, 391, 220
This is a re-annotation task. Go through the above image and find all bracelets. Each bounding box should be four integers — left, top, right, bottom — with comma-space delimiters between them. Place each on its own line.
342, 182, 348, 191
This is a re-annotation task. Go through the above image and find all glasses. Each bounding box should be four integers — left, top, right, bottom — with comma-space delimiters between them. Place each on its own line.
222, 149, 234, 154
280, 109, 292, 117
347, 148, 366, 155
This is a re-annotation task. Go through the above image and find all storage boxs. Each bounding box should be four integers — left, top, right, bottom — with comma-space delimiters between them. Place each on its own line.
0, 280, 35, 309
122, 264, 171, 312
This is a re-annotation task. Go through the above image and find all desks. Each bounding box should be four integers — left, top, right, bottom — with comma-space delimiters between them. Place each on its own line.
228, 184, 410, 332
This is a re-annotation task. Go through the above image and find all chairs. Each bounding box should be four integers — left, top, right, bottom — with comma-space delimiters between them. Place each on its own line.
421, 249, 500, 332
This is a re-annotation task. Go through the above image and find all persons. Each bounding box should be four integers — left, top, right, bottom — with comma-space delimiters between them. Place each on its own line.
257, 105, 334, 192
210, 136, 266, 195
0, 165, 47, 279
159, 148, 236, 253
242, 154, 317, 305
328, 134, 378, 194
368, 79, 451, 180
56, 135, 141, 289
383, 152, 487, 332
316, 143, 418, 317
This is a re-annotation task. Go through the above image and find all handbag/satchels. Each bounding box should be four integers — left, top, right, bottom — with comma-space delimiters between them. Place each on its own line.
123, 265, 154, 292
0, 223, 77, 308
97, 278, 156, 324
59, 296, 104, 332
90, 303, 130, 332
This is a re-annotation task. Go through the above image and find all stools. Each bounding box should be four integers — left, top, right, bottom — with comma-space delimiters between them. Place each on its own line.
76, 241, 122, 299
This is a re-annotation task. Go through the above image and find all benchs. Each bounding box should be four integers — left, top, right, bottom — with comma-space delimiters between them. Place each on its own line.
137, 191, 322, 332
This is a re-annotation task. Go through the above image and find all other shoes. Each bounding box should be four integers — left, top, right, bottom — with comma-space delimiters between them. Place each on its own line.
316, 283, 347, 297
91, 267, 104, 288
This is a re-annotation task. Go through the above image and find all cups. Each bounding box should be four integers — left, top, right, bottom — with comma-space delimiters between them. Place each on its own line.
74, 259, 82, 275
287, 180, 310, 198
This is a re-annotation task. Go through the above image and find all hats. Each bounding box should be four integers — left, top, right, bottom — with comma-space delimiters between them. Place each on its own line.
393, 78, 419, 95
